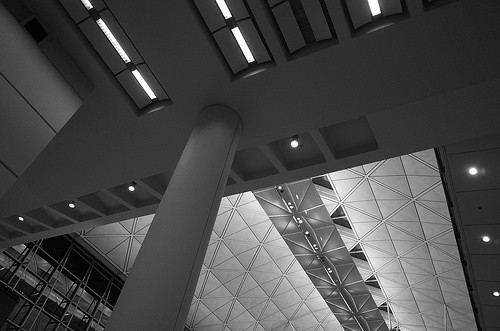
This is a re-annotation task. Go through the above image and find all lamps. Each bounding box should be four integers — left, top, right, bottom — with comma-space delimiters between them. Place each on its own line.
128, 181, 136, 191
69, 201, 75, 208
290, 134, 299, 148
18, 216, 24, 221
